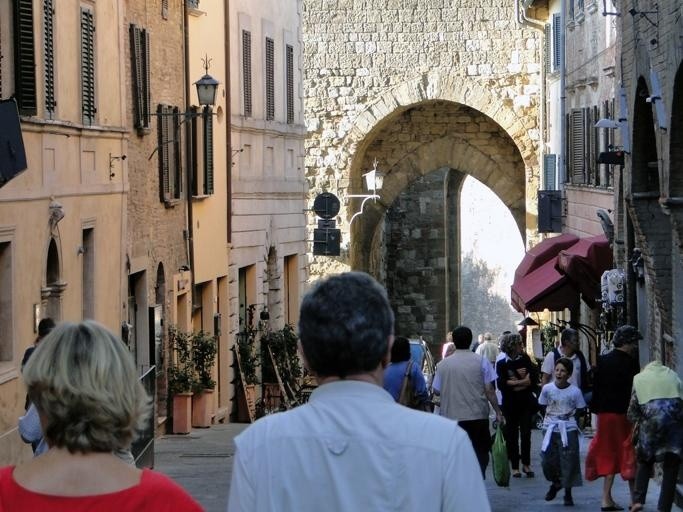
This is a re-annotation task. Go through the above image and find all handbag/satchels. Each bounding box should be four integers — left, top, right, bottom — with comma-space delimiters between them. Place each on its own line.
491, 422, 511, 487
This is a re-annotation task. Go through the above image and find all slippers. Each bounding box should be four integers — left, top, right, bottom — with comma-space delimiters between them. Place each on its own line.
600, 502, 625, 512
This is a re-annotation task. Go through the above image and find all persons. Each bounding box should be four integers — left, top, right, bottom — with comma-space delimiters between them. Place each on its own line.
543, 329, 592, 402
20, 316, 59, 456
587, 324, 644, 511
629, 361, 683, 512
433, 327, 503, 480
227, 271, 491, 512
471, 334, 485, 353
496, 333, 540, 477
442, 331, 455, 357
384, 334, 434, 411
538, 359, 587, 505
475, 331, 500, 366
1, 321, 204, 511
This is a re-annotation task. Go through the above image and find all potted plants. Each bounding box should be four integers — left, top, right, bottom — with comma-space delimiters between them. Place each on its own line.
187, 330, 221, 427
258, 327, 284, 414
167, 323, 199, 433
234, 325, 264, 422
279, 323, 303, 412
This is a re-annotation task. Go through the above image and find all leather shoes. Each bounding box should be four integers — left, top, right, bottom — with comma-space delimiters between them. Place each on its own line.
545, 483, 563, 501
564, 496, 573, 506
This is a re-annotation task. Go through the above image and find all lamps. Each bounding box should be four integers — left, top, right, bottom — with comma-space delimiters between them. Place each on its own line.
362, 157, 384, 190
192, 55, 218, 104
